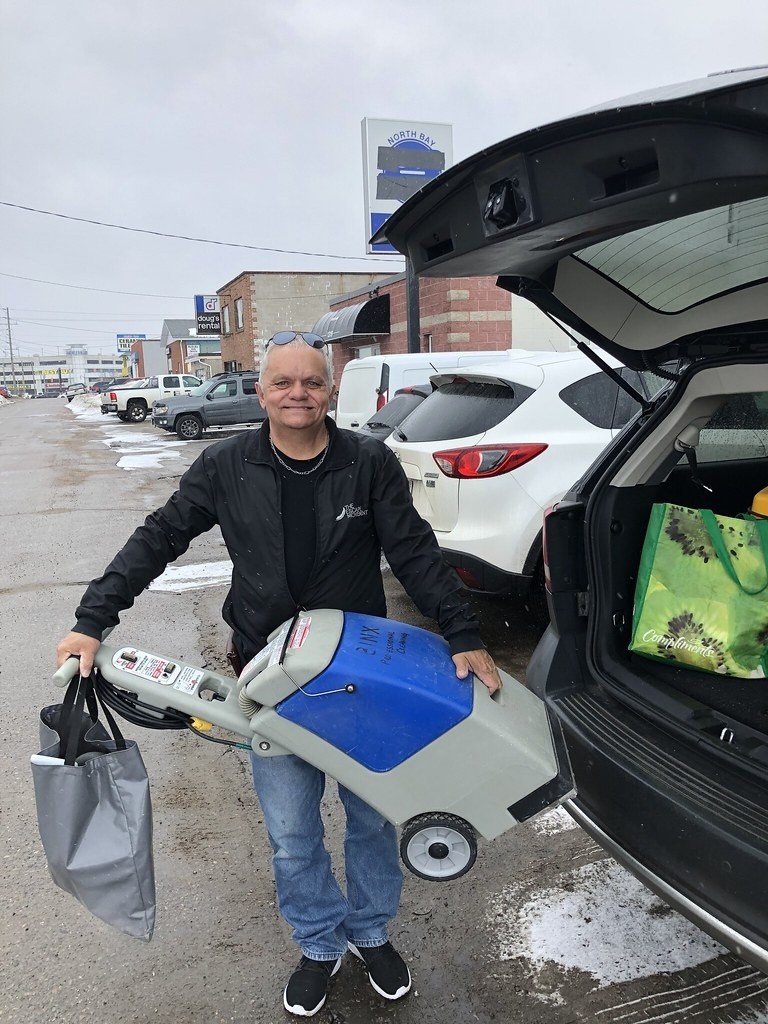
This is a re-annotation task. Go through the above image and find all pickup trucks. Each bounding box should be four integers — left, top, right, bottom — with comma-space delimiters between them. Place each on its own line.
100, 374, 226, 423
66, 383, 88, 402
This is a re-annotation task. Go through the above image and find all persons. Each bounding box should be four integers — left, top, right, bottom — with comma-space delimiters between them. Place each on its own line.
58, 332, 500, 1016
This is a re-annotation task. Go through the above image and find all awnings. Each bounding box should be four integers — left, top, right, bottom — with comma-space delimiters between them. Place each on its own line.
312, 294, 390, 343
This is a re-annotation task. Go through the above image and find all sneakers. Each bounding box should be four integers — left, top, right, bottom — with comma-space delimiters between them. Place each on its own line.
283, 954, 341, 1016
347, 940, 412, 1000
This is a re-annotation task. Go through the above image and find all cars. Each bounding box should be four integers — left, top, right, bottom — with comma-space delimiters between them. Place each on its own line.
89, 377, 188, 392
23, 392, 66, 398
368, 63, 768, 978
0, 386, 12, 398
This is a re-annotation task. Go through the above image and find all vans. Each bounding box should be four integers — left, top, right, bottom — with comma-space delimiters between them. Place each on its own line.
332, 349, 550, 433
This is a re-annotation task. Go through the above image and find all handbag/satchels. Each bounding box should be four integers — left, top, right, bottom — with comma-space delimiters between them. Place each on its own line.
628, 503, 768, 678
31, 671, 156, 942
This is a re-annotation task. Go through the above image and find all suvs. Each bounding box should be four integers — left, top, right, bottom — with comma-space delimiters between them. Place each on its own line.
349, 351, 768, 628
151, 369, 269, 440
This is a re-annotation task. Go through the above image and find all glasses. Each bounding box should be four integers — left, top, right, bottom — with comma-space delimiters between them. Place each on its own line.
265, 331, 329, 356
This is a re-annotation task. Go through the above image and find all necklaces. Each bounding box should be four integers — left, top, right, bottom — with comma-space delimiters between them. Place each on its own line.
269, 435, 329, 474
